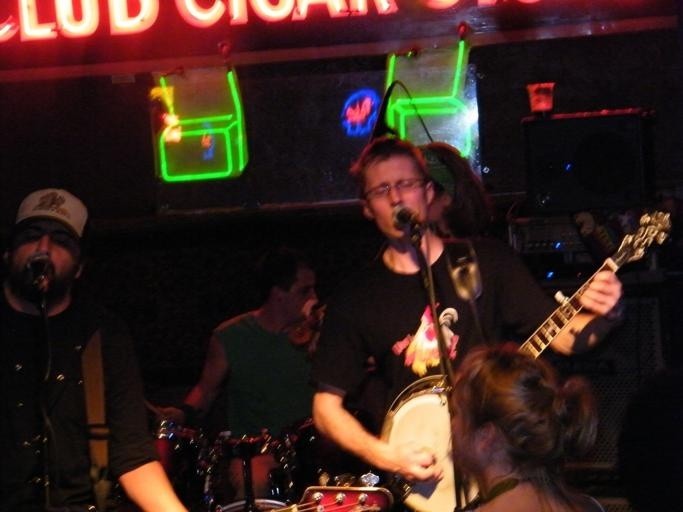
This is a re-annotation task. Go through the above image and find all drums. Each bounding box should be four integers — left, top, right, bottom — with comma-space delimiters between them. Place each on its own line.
214, 430, 294, 509
150, 420, 221, 506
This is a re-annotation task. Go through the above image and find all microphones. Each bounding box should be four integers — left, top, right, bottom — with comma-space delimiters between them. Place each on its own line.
391, 204, 424, 226
24, 253, 56, 310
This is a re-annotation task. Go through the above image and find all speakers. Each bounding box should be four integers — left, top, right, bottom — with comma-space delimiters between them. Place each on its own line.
524, 116, 657, 212
540, 271, 683, 512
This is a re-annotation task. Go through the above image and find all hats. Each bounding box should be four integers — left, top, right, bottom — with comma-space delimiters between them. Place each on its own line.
14, 188, 97, 255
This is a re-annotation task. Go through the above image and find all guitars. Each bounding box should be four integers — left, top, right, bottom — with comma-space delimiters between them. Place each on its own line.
274, 473, 394, 511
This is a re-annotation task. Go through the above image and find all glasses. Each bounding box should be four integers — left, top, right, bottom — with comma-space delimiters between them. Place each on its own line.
363, 177, 423, 197
